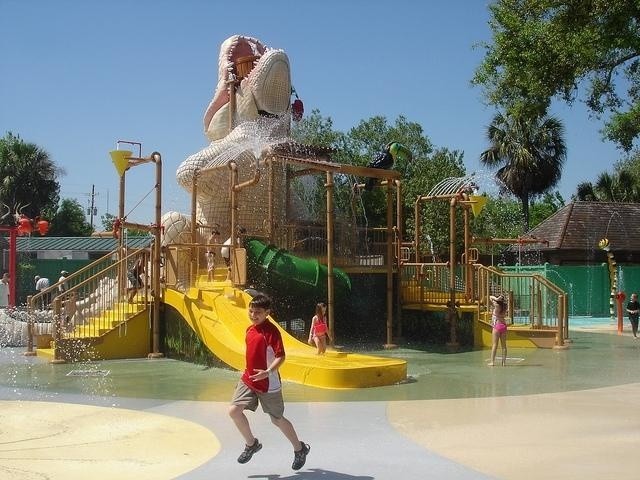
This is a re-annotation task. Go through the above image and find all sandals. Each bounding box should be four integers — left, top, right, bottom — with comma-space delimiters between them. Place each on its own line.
237, 438, 263, 464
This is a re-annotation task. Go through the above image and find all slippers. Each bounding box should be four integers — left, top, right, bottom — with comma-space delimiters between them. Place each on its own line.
291, 440, 311, 471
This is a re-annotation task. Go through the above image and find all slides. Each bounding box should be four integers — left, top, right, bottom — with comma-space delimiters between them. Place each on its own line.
165, 278, 407, 388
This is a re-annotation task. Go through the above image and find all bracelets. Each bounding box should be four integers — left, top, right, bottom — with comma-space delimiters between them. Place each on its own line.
310, 334, 313, 336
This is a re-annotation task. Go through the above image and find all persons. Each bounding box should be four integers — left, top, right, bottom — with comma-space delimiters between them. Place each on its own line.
221, 227, 248, 278
626, 293, 640, 338
308, 303, 333, 355
0, 272, 10, 307
206, 229, 219, 280
35, 276, 51, 309
488, 295, 507, 366
124, 250, 151, 304
229, 296, 309, 470
58, 270, 69, 293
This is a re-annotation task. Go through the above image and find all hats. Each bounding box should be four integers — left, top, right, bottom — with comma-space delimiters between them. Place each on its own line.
34, 275, 41, 283
61, 271, 69, 276
